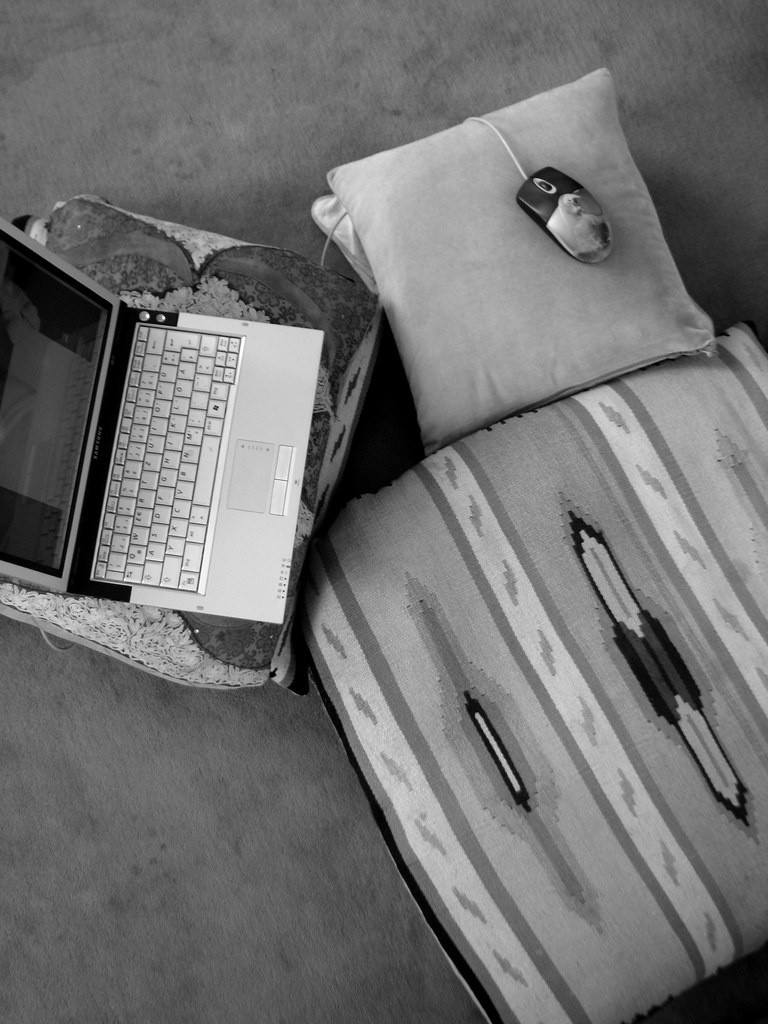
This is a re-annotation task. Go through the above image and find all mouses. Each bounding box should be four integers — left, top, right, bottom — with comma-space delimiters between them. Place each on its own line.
516, 165, 614, 265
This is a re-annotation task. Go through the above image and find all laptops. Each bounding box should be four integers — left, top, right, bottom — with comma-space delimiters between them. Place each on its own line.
0, 216, 325, 623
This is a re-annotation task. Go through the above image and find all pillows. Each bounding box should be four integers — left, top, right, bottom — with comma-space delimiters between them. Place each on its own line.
319, 64, 719, 463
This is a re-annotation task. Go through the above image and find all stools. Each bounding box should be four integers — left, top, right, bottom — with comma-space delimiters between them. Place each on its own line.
0, 188, 392, 698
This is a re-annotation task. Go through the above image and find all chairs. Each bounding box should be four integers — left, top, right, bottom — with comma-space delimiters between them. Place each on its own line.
292, 312, 768, 1024
